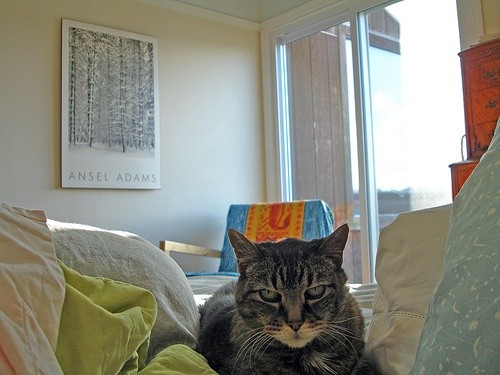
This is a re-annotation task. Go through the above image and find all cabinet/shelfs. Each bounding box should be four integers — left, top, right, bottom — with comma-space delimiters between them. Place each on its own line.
448, 38, 499, 203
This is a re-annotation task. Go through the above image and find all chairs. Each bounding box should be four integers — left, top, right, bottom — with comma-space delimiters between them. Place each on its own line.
158, 199, 334, 279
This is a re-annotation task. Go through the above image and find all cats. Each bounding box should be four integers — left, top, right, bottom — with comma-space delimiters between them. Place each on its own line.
196, 223, 377, 375
